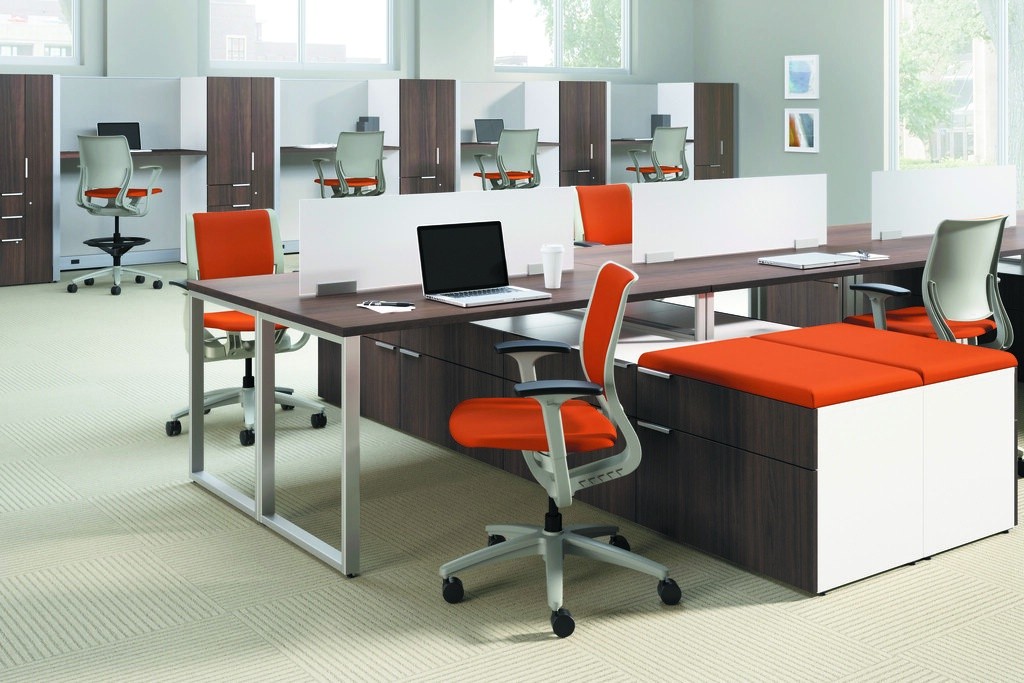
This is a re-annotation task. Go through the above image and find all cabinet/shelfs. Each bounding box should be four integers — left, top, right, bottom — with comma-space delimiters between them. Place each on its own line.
693, 82, 734, 180
207, 76, 275, 213
317, 258, 1024, 600
0, 74, 54, 288
399, 78, 456, 195
559, 80, 607, 187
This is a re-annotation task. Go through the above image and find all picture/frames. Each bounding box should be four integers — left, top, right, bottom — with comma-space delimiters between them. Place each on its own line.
784, 108, 820, 154
784, 54, 819, 100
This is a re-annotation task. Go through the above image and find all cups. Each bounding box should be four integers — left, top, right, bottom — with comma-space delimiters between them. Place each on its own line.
541, 244, 564, 288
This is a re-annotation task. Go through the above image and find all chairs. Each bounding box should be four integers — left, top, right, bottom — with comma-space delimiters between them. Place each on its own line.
625, 127, 689, 183
66, 134, 164, 297
437, 259, 688, 643
311, 131, 388, 199
473, 128, 540, 191
841, 214, 1024, 481
573, 183, 632, 247
164, 208, 329, 448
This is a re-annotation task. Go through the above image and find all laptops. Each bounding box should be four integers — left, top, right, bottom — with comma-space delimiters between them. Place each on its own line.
97, 122, 152, 152
416, 221, 552, 308
757, 252, 860, 269
474, 119, 505, 144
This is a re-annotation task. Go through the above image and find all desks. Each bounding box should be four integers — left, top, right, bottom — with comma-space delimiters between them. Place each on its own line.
611, 138, 694, 145
281, 145, 400, 152
187, 225, 1024, 582
60, 148, 207, 159
461, 141, 559, 148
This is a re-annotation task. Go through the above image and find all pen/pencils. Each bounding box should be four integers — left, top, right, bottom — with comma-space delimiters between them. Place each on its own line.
362, 300, 415, 307
